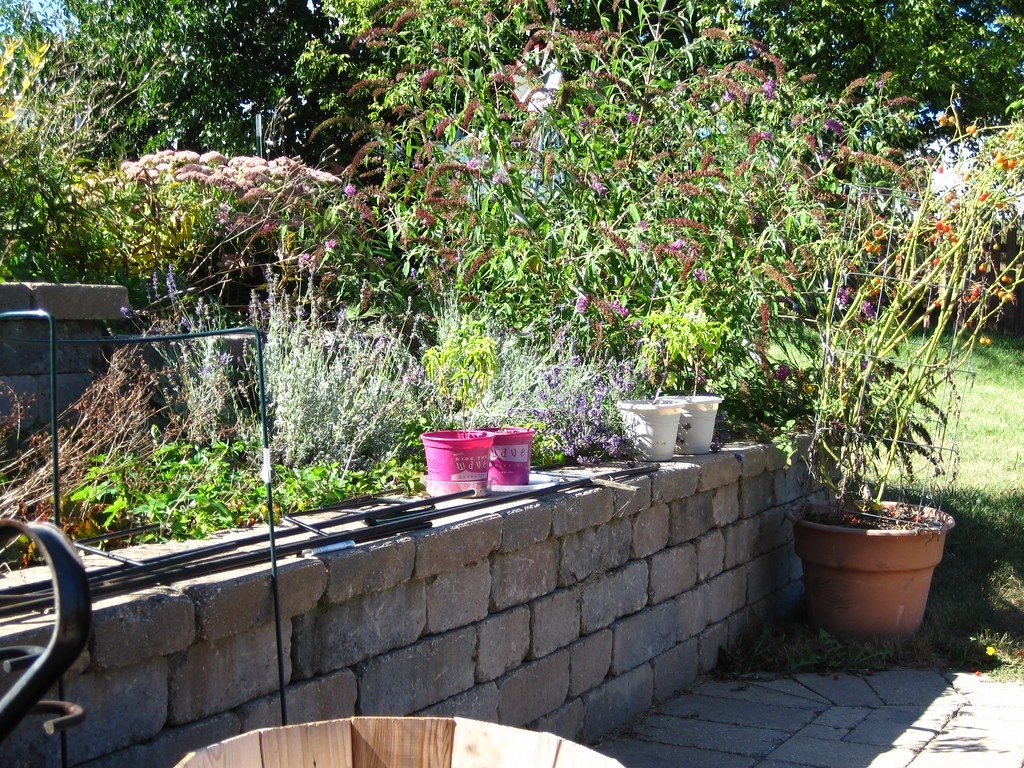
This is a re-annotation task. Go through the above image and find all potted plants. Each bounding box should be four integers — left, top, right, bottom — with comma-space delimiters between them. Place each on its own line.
419, 314, 536, 497
618, 301, 723, 461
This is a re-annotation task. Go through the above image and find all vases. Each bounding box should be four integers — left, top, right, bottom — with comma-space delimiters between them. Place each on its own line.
794, 500, 955, 640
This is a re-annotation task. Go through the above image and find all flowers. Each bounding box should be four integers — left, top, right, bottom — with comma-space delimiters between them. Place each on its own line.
770, 83, 1024, 514
